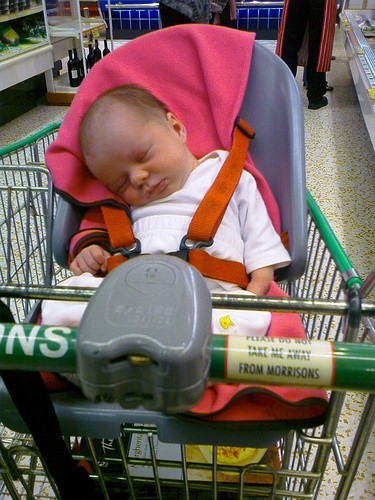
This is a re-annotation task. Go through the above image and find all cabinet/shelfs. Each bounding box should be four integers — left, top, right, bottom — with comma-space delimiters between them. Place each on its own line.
0, 0, 114, 129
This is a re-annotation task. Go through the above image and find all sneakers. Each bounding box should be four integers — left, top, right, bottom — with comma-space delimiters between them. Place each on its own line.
308, 96, 328, 109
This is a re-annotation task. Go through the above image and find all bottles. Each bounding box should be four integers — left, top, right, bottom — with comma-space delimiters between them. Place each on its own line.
67, 39, 110, 88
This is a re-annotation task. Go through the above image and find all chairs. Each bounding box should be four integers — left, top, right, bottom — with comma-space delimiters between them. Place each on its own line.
52, 25, 307, 284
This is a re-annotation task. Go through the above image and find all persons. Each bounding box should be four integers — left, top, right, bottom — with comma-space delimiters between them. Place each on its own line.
159, 0, 238, 31
302, 64, 334, 91
274, 0, 337, 110
41, 83, 291, 339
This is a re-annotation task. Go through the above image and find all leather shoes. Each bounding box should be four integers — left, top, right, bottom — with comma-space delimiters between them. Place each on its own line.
306, 84, 334, 91
303, 81, 328, 86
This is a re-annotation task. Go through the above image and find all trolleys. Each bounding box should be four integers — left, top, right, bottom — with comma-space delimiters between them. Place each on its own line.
0, 112, 375, 500
